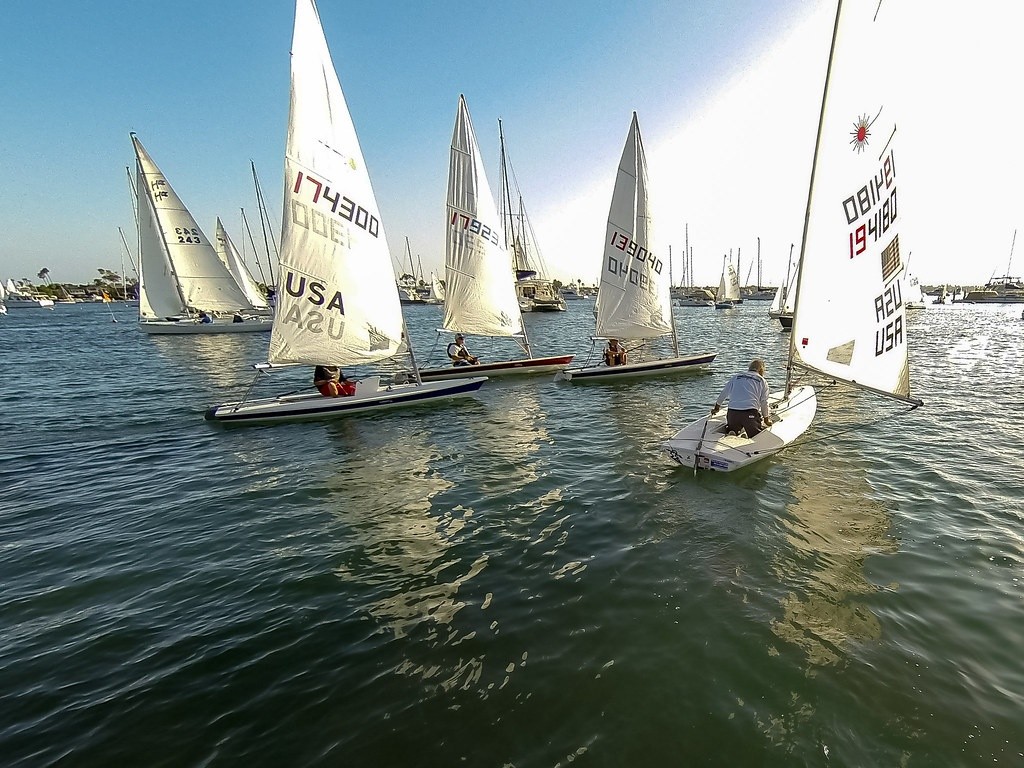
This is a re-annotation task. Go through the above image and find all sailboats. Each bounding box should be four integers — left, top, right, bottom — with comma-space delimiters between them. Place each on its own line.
904, 252, 926, 310
392, 235, 446, 306
564, 109, 717, 380
164, 216, 274, 320
659, 0, 924, 470
205, 0, 491, 425
53, 226, 141, 303
5, 295, 55, 308
129, 131, 277, 335
679, 220, 800, 331
493, 115, 570, 313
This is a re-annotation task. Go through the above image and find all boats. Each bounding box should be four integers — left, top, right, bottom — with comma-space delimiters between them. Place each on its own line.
952, 230, 1024, 303
925, 287, 950, 296
932, 295, 945, 304
953, 286, 962, 294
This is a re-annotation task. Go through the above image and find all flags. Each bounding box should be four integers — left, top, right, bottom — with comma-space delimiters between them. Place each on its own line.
102, 291, 111, 302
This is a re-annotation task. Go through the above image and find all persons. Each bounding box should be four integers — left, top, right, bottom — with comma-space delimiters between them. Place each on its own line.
711, 360, 773, 439
198, 310, 210, 324
603, 339, 627, 367
448, 333, 480, 367
314, 366, 356, 398
232, 312, 244, 324
